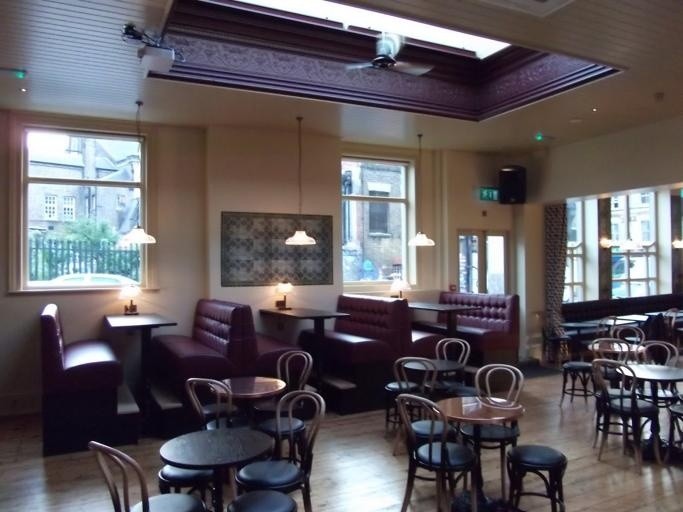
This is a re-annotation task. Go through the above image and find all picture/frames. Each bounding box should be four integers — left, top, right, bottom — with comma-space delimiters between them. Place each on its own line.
221, 211, 334, 288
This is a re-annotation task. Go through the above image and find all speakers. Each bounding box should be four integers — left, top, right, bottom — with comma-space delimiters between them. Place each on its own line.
498, 166, 527, 205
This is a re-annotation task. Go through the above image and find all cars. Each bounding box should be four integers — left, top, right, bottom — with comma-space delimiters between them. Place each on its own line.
48, 273, 137, 284
611, 255, 636, 290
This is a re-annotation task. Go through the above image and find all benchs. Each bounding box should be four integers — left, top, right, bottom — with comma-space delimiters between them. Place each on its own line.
145, 297, 321, 435
412, 290, 520, 393
39, 302, 140, 457
299, 292, 448, 416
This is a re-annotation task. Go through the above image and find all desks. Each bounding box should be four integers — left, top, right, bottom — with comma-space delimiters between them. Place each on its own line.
105, 311, 177, 437
260, 304, 349, 383
407, 299, 481, 359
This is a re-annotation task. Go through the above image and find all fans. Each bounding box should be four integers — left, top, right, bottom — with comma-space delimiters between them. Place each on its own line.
343, 30, 434, 79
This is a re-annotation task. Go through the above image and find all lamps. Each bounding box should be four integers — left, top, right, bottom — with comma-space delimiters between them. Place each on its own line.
123, 23, 186, 76
117, 282, 141, 315
390, 279, 412, 298
408, 134, 435, 247
117, 102, 157, 245
275, 281, 295, 311
284, 116, 316, 246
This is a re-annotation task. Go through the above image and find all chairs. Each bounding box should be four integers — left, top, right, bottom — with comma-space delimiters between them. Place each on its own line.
88, 347, 326, 511
385, 337, 526, 512
559, 306, 682, 475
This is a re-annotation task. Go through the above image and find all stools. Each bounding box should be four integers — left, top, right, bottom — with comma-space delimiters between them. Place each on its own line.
507, 446, 567, 512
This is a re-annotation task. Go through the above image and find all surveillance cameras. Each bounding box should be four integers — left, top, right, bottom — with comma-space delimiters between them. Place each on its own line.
121, 23, 144, 44
